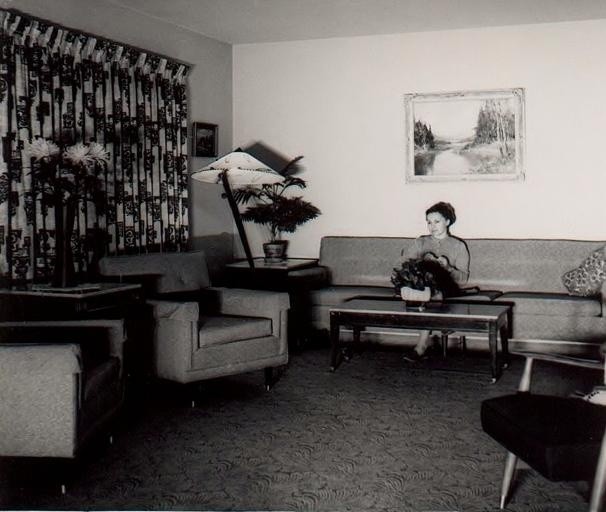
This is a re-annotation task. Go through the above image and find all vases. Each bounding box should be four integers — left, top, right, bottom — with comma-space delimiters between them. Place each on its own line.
53, 203, 78, 287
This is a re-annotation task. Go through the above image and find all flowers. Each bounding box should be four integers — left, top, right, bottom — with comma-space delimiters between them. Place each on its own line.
23, 138, 109, 214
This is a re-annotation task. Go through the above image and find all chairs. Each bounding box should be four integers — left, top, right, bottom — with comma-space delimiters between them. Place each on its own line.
0, 320, 127, 493
99, 250, 290, 410
481, 339, 606, 512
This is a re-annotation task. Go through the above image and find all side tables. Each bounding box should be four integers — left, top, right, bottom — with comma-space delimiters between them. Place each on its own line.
0, 282, 143, 434
227, 258, 317, 355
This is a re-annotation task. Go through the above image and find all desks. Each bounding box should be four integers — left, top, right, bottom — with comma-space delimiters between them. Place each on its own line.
327, 298, 511, 384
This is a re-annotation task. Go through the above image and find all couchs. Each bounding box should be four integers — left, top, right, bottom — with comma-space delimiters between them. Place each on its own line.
288, 235, 606, 361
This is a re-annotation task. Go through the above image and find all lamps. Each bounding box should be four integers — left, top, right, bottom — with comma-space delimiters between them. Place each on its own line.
190, 147, 286, 274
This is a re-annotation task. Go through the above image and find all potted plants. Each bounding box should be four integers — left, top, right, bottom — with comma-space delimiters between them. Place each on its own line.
392, 259, 441, 307
221, 156, 321, 265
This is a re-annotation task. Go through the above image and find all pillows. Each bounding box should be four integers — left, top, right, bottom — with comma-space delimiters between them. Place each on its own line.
561, 244, 606, 298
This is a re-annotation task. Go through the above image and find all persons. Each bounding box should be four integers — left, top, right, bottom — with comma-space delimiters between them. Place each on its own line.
392, 201, 470, 361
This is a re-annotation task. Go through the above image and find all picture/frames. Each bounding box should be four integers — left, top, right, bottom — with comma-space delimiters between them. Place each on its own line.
404, 88, 526, 183
192, 121, 219, 157
